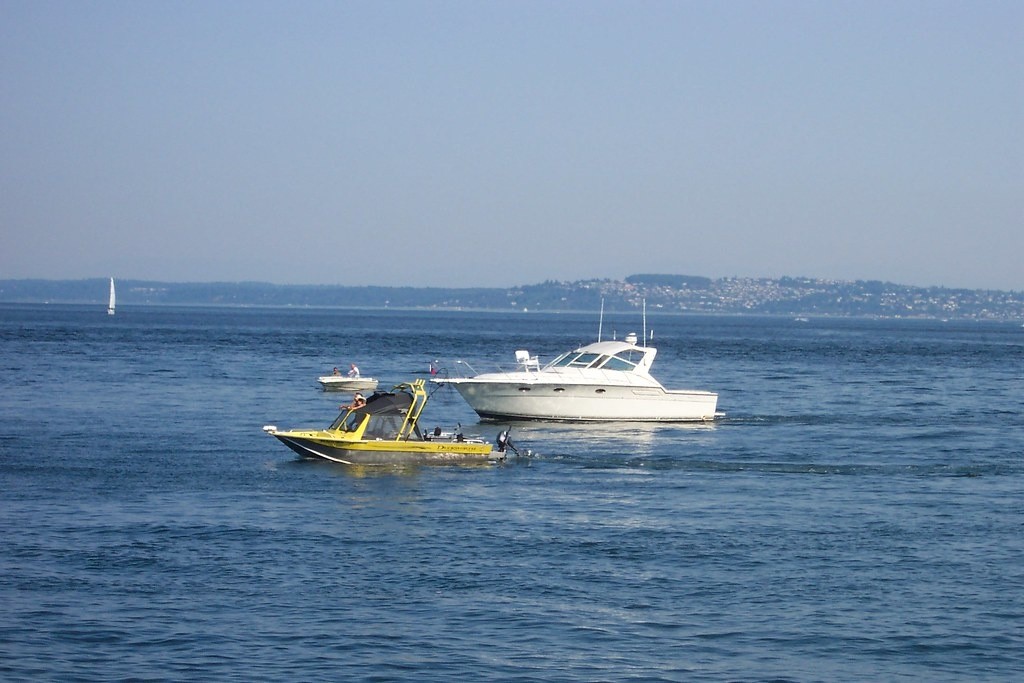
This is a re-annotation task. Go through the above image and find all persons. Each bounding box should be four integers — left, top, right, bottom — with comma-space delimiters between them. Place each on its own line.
333, 367, 340, 376
348, 363, 359, 378
343, 392, 367, 438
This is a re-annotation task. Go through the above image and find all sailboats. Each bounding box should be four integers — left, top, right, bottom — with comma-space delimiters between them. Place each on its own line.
105, 277, 116, 315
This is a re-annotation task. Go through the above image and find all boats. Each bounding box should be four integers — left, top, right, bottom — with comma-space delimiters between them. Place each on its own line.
261, 378, 523, 465
316, 377, 379, 391
428, 295, 728, 422
